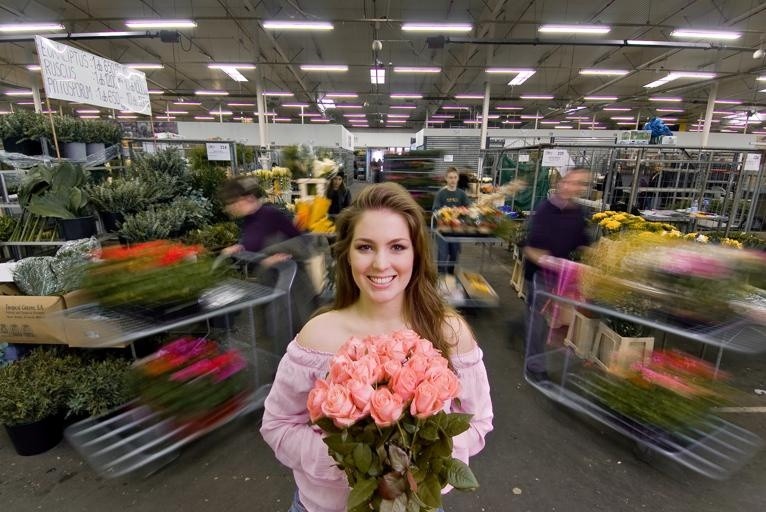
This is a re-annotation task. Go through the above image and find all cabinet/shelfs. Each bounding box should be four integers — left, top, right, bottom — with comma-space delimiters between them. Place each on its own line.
383, 157, 444, 229
290, 177, 329, 206
353, 149, 366, 182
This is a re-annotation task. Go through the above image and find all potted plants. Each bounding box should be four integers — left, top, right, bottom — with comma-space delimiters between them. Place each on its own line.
24, 161, 97, 241
88, 176, 147, 232
116, 209, 172, 246
0, 350, 81, 456
0, 110, 120, 165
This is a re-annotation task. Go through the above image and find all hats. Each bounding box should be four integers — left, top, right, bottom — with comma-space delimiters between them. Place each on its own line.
218, 176, 258, 199
337, 168, 344, 178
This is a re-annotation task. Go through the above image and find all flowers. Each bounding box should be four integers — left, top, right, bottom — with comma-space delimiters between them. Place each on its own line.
591, 208, 744, 250
575, 306, 600, 319
305, 328, 480, 512
241, 166, 293, 206
433, 205, 502, 226
605, 293, 650, 336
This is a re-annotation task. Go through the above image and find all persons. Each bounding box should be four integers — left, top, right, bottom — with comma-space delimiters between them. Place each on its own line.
218, 176, 320, 382
516, 166, 596, 390
271, 162, 279, 183
627, 165, 647, 210
457, 169, 472, 190
432, 166, 473, 275
370, 157, 383, 174
601, 163, 624, 211
326, 169, 352, 258
258, 181, 494, 512
645, 161, 664, 209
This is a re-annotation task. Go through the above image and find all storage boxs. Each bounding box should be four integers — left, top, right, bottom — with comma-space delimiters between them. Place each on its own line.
616, 130, 651, 146
63, 309, 134, 348
0, 284, 96, 344
655, 134, 677, 145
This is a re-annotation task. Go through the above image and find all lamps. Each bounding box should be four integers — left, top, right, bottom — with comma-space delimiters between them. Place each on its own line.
261, 19, 335, 33
670, 30, 742, 41
2, 62, 765, 134
0, 23, 66, 32
124, 18, 197, 28
537, 24, 612, 34
399, 22, 474, 32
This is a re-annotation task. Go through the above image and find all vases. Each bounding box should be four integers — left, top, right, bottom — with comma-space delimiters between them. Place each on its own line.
437, 225, 495, 237
563, 306, 600, 358
589, 320, 656, 378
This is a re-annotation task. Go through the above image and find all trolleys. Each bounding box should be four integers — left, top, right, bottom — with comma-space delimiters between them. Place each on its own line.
425, 207, 505, 316
39, 246, 300, 482
519, 244, 766, 481
278, 213, 342, 313
